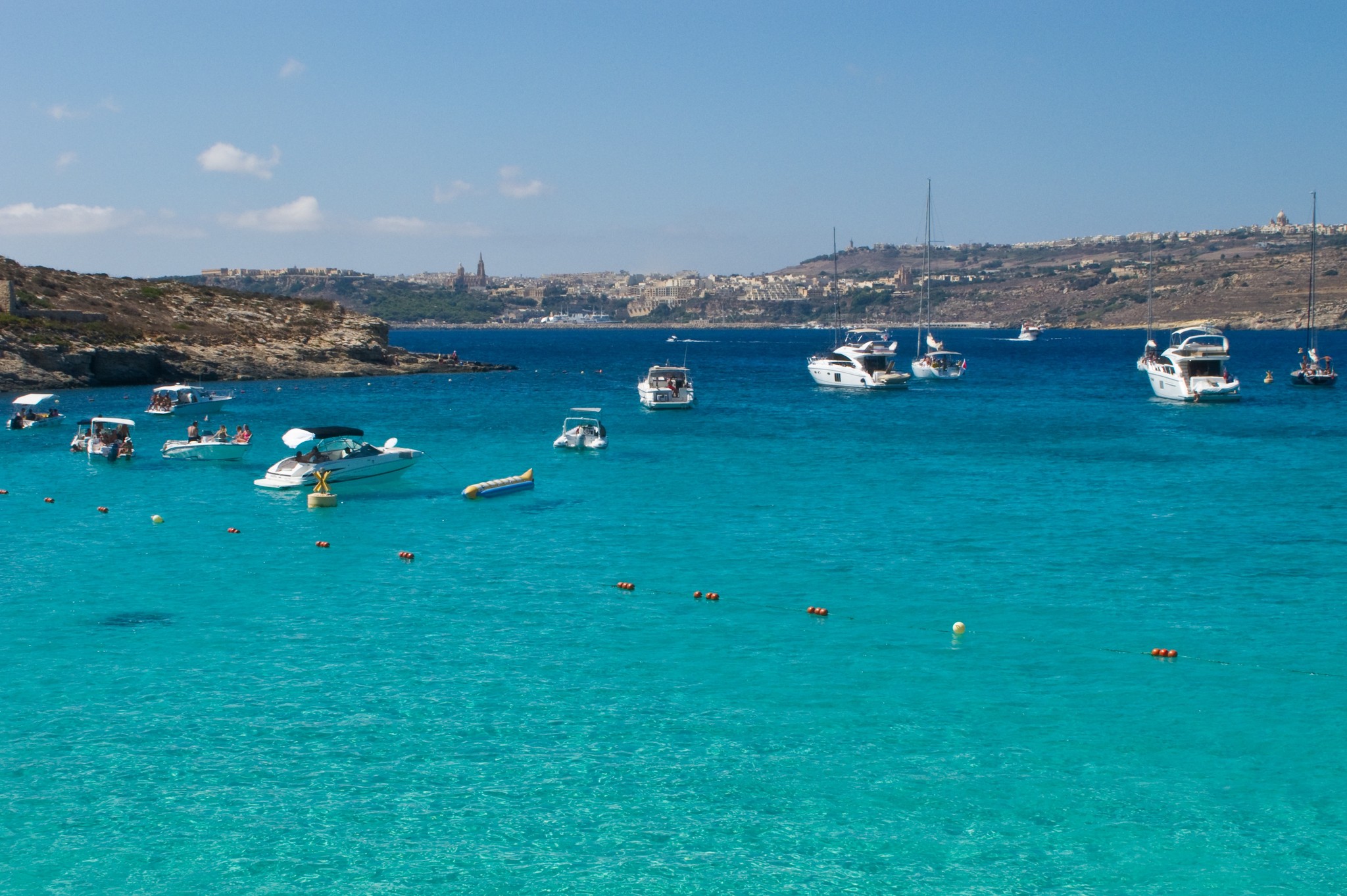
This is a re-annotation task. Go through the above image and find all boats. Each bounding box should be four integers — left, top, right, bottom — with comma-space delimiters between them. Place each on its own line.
638, 359, 693, 409
8, 394, 66, 429
1136, 231, 1244, 401
145, 385, 233, 415
1017, 322, 1041, 341
254, 427, 423, 489
162, 430, 252, 460
71, 417, 134, 457
667, 335, 677, 341
552, 407, 609, 449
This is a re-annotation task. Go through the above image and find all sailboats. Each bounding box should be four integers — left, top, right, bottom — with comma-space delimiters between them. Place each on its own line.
912, 179, 966, 379
807, 226, 911, 389
1289, 192, 1339, 385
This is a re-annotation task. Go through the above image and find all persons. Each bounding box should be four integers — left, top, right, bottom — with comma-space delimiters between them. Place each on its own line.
1196, 370, 1220, 376
295, 446, 352, 464
1301, 354, 1332, 374
682, 375, 689, 388
921, 353, 963, 367
576, 425, 595, 434
1193, 390, 1199, 403
209, 425, 251, 442
187, 420, 199, 444
19, 408, 58, 421
595, 421, 607, 437
1142, 351, 1160, 364
148, 392, 217, 412
70, 415, 133, 457
1226, 372, 1233, 383
667, 376, 678, 397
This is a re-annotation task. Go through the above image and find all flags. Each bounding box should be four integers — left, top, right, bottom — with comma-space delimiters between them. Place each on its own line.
962, 361, 967, 370
1224, 370, 1227, 379
